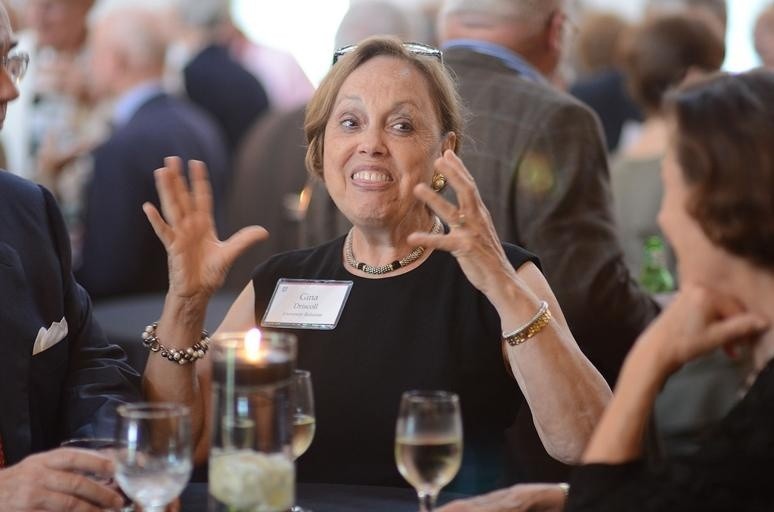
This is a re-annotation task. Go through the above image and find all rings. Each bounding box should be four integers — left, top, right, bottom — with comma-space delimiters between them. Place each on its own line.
449, 212, 465, 230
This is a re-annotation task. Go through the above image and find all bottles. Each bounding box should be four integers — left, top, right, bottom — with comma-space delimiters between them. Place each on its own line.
636, 236, 679, 310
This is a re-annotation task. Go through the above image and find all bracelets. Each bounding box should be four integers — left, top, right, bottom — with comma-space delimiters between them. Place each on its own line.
140, 321, 210, 366
501, 300, 551, 346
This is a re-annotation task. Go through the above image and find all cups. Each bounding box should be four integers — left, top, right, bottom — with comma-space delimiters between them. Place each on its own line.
394, 389, 463, 512
111, 402, 195, 512
295, 370, 315, 461
59, 439, 135, 512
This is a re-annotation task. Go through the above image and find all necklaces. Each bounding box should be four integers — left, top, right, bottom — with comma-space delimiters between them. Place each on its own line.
344, 215, 441, 274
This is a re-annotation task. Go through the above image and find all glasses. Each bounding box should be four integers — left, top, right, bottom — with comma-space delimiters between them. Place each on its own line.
331, 42, 443, 61
2, 51, 33, 78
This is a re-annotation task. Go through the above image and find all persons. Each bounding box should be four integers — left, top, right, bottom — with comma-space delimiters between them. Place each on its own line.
140, 34, 615, 495
164, 0, 270, 150
434, 71, 773, 512
436, 1, 663, 385
738, 0, 774, 108
231, 2, 417, 294
565, 12, 644, 151
6, 3, 103, 270
194, 1, 313, 115
76, 2, 223, 299
612, 16, 725, 292
0, 2, 142, 512
